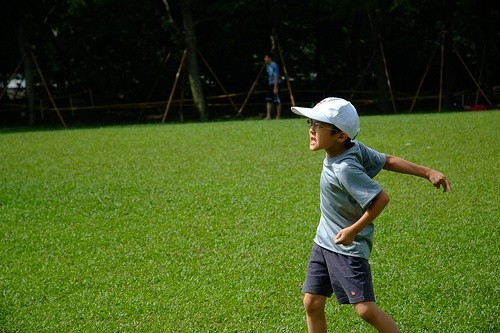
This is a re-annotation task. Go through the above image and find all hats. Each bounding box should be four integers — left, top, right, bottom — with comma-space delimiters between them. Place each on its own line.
290, 97, 360, 140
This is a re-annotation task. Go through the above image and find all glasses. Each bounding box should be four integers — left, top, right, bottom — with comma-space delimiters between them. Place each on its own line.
306, 118, 340, 136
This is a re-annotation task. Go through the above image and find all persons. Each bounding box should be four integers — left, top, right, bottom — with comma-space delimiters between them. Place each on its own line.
290, 96, 451, 333
262, 51, 282, 120
